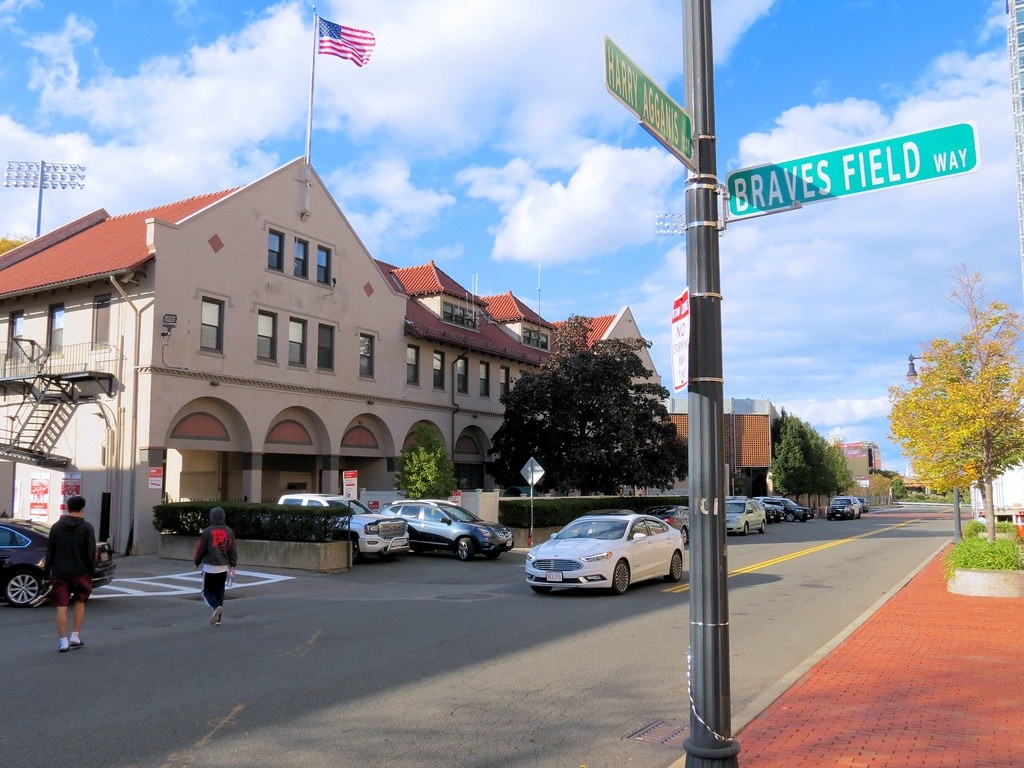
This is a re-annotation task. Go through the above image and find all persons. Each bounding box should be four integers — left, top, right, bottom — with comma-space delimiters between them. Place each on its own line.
41, 495, 97, 654
193, 506, 238, 627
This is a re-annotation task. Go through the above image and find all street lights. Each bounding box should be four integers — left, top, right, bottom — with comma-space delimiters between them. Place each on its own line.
906, 353, 966, 544
4, 160, 86, 237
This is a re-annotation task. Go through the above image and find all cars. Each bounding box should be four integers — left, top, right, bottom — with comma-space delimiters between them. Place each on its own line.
725, 496, 767, 535
0, 519, 117, 608
858, 498, 869, 513
525, 514, 685, 595
584, 505, 689, 544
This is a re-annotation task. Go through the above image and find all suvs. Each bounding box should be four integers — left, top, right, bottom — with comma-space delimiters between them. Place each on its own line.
827, 495, 862, 520
379, 500, 515, 561
277, 494, 410, 565
753, 497, 814, 523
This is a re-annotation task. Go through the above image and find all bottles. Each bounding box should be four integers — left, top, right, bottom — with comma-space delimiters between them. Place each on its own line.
227, 566, 234, 587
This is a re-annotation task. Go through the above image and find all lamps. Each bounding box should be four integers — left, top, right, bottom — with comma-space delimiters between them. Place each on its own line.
120, 271, 139, 284
323, 277, 336, 296
161, 311, 177, 337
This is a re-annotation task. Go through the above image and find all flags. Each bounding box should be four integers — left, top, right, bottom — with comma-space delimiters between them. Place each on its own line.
316, 16, 376, 67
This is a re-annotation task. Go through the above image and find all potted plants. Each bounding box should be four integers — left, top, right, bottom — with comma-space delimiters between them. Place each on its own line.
941, 518, 1024, 598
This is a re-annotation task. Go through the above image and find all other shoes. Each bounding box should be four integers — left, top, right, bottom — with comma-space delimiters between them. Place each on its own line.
210, 605, 223, 625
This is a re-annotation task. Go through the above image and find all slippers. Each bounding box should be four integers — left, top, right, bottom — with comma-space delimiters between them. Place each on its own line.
69, 639, 84, 646
59, 645, 69, 652
